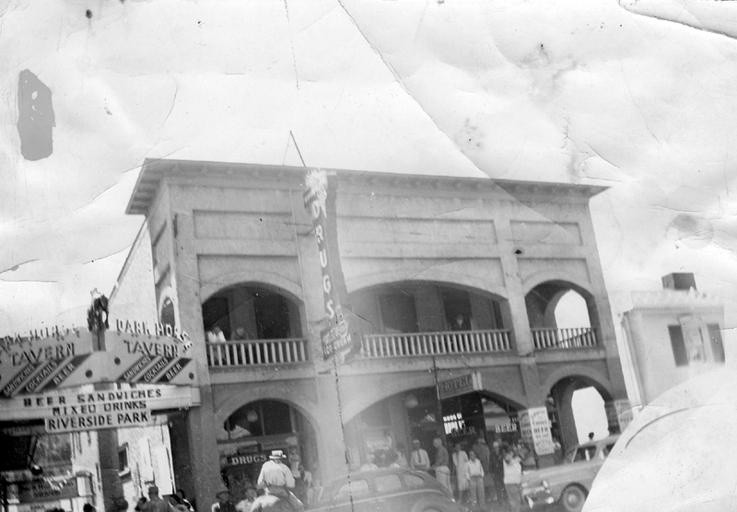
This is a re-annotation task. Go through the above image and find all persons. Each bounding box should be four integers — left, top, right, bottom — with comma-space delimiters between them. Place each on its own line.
10, 446, 313, 511
228, 325, 250, 364
205, 321, 227, 365
357, 428, 595, 511
444, 304, 479, 351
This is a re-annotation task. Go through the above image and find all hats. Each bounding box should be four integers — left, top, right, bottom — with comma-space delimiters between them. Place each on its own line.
268, 449, 287, 460
